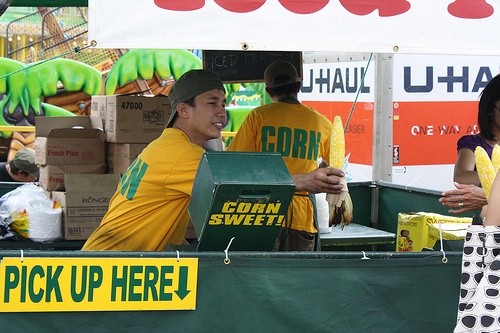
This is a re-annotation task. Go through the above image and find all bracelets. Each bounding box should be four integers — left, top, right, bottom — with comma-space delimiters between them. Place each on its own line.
482, 216, 487, 226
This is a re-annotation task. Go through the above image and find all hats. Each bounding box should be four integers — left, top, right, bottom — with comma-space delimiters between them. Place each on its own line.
264, 60, 301, 88
13, 148, 40, 178
163, 70, 227, 129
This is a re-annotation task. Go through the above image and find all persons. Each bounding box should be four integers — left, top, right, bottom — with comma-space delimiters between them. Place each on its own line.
439, 75, 500, 226
80, 70, 345, 251
0, 148, 39, 182
230, 61, 334, 251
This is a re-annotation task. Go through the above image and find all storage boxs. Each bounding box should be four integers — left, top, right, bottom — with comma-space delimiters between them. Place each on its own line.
396, 211, 471, 259
36, 89, 172, 241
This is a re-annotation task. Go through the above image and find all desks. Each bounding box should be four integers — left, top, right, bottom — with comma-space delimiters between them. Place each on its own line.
320, 223, 399, 251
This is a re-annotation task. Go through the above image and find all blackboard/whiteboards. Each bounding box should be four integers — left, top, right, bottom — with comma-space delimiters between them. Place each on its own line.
202, 47, 303, 84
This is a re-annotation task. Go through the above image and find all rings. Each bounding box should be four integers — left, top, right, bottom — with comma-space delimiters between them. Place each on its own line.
458, 202, 464, 209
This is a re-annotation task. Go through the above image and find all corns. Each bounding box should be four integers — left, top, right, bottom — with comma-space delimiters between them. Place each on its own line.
474, 144, 500, 201
329, 115, 344, 170
